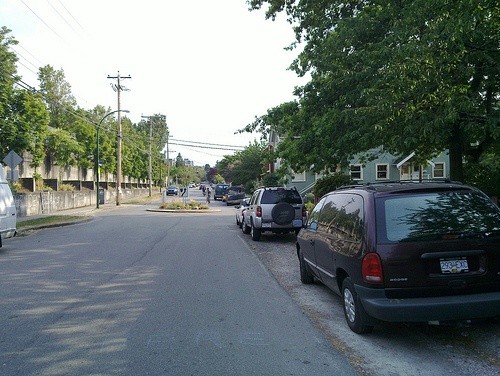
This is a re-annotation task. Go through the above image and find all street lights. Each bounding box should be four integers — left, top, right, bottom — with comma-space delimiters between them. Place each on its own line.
96, 109, 129, 208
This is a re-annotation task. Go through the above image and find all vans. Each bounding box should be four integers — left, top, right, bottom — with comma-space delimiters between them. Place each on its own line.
295, 178, 500, 336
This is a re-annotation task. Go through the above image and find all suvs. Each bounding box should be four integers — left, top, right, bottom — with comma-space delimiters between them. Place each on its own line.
243, 186, 309, 244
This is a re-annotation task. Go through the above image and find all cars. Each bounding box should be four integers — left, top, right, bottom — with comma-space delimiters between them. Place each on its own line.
226, 186, 245, 206
235, 197, 251, 224
213, 184, 230, 201
166, 185, 177, 196
1, 176, 17, 248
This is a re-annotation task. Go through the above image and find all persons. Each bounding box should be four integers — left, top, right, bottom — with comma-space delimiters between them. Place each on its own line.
200, 184, 207, 196
207, 188, 211, 203
